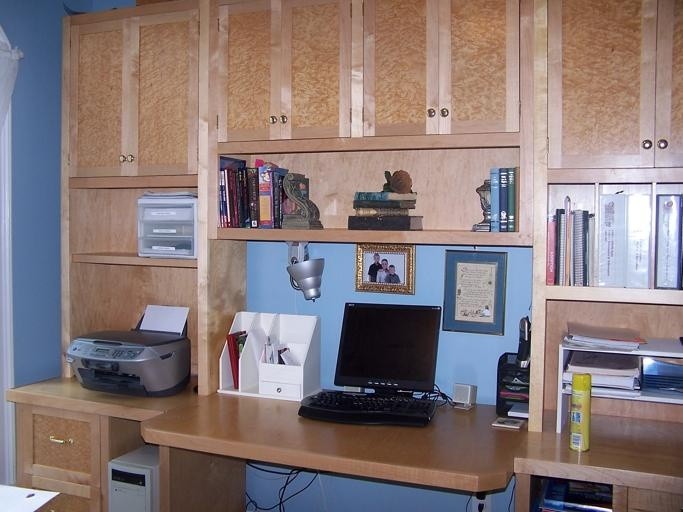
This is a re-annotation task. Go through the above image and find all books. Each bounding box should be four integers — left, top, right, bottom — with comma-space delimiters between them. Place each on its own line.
218, 157, 310, 228
549, 194, 680, 290
343, 191, 424, 229
545, 481, 615, 512
561, 325, 682, 397
490, 168, 518, 232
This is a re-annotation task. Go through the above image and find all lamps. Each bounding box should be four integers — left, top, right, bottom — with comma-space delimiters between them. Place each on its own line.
286, 241, 326, 301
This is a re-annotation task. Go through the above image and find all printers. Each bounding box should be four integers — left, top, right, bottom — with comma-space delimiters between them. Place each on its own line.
66, 304, 192, 398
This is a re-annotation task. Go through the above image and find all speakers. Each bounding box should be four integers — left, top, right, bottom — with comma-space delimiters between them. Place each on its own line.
449, 383, 478, 411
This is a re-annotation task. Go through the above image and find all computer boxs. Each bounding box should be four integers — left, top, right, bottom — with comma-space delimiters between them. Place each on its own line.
109, 444, 160, 512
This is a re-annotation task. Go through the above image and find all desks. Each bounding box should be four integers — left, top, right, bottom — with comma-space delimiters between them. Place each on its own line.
141, 388, 527, 512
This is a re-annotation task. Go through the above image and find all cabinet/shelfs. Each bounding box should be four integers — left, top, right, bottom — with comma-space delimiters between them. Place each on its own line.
209, 0, 362, 143
512, 171, 683, 512
207, 133, 534, 248
7, 376, 246, 511
61, 0, 208, 178
60, 174, 247, 394
363, 0, 534, 138
534, 0, 682, 169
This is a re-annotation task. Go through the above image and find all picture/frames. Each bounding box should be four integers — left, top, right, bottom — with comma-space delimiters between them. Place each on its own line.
355, 243, 416, 296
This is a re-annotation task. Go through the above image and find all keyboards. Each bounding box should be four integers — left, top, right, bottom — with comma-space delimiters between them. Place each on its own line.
298, 388, 437, 428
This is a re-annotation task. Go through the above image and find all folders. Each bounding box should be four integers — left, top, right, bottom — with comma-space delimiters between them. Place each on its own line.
654, 194, 683, 291
216, 311, 323, 404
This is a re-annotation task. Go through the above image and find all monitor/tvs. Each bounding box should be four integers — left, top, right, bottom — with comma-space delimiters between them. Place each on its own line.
333, 302, 443, 393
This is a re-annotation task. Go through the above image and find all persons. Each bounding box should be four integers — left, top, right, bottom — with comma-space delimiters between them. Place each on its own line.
376, 259, 389, 284
386, 265, 400, 283
368, 254, 381, 282
